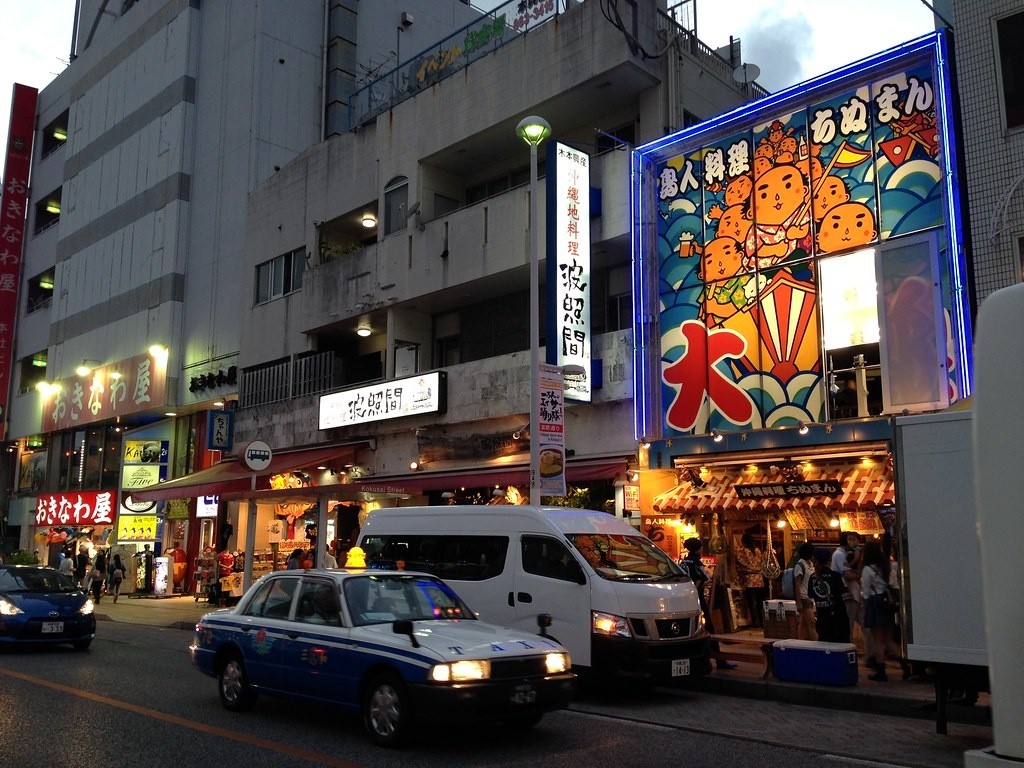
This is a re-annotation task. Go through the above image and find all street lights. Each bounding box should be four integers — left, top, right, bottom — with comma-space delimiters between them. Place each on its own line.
515, 114, 552, 508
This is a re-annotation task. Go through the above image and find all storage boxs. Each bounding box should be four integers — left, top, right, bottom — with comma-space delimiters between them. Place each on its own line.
773, 639, 859, 686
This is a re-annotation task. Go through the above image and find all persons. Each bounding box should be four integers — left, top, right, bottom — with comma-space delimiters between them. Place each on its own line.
304, 586, 337, 625
72, 545, 92, 588
838, 378, 858, 405
830, 531, 888, 681
90, 549, 108, 605
735, 534, 765, 630
794, 542, 818, 640
323, 544, 337, 568
108, 554, 126, 604
678, 538, 738, 670
33, 551, 45, 564
807, 550, 851, 644
59, 550, 76, 582
218, 549, 234, 606
285, 549, 304, 570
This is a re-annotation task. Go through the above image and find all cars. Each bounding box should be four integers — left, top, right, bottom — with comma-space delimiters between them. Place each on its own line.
0, 563, 97, 653
186, 567, 581, 749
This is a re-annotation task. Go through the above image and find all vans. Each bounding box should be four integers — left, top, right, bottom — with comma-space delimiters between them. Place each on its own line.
342, 502, 721, 708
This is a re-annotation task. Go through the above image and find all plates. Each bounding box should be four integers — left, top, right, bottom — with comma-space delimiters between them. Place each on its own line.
538, 445, 564, 479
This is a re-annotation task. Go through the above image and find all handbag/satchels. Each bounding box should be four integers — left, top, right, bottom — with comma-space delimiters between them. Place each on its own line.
872, 585, 900, 613
91, 569, 100, 577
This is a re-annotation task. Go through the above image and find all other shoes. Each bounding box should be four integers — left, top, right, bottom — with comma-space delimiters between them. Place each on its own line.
114, 595, 118, 603
871, 663, 886, 675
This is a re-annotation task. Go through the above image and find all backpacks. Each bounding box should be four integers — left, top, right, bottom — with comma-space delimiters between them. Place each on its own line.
113, 564, 122, 584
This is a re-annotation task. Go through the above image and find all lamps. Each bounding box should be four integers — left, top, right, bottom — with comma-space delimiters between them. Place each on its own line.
712, 427, 749, 443
798, 421, 832, 435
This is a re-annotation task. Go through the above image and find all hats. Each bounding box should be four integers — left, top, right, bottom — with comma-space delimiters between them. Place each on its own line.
861, 541, 882, 557
685, 538, 702, 552
